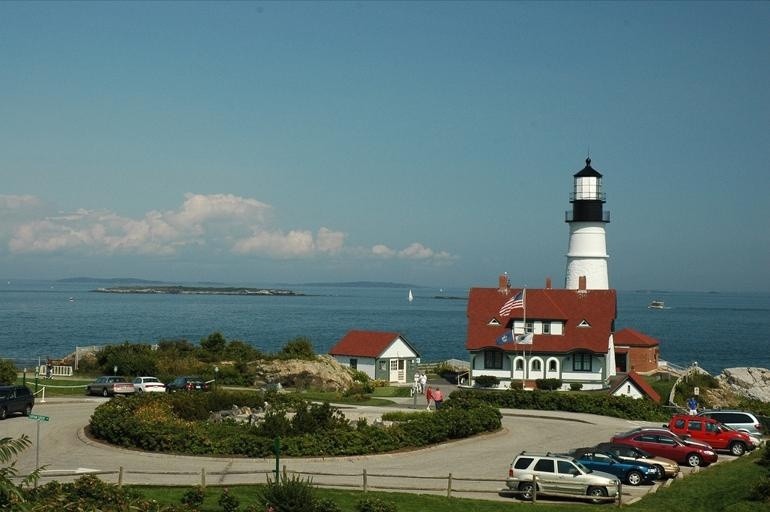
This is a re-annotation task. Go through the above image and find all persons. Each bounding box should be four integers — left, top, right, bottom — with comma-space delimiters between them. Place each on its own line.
426, 386, 434, 412
418, 373, 427, 394
688, 396, 698, 416
433, 388, 443, 411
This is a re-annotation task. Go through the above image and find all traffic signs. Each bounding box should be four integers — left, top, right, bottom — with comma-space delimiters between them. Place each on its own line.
27, 412, 50, 422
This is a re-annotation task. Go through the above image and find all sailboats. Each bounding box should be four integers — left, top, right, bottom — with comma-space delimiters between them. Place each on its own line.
407, 288, 415, 303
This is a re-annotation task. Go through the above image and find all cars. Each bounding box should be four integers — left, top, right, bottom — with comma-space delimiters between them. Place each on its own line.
164, 375, 212, 393
595, 441, 682, 482
131, 375, 169, 394
609, 425, 720, 469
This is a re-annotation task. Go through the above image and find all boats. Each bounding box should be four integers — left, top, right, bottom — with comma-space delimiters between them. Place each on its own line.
647, 301, 665, 309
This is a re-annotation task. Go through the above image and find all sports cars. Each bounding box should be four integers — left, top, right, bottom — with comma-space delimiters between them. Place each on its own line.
543, 445, 660, 487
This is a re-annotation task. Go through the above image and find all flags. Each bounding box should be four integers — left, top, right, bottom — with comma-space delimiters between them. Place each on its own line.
496, 330, 514, 346
515, 333, 533, 345
499, 289, 524, 317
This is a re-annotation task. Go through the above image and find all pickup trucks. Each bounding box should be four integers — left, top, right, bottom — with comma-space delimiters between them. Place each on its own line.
83, 374, 136, 398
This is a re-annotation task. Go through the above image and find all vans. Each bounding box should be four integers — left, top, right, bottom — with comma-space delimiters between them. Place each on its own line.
693, 409, 764, 440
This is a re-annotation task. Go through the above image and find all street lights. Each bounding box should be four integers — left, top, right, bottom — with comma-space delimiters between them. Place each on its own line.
34, 365, 39, 397
21, 367, 26, 385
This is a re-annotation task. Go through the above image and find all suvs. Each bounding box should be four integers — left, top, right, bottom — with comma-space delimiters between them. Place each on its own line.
665, 413, 762, 458
0, 385, 36, 420
504, 448, 621, 507
